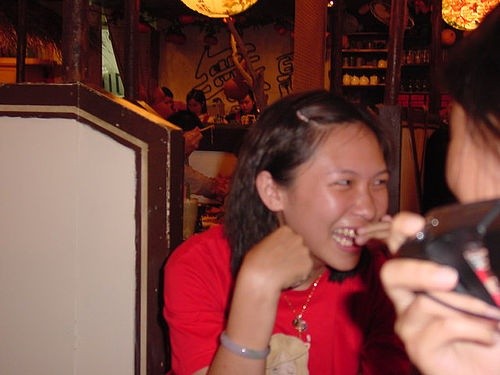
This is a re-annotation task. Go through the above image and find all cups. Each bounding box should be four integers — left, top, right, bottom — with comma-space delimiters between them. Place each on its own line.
183, 198, 198, 240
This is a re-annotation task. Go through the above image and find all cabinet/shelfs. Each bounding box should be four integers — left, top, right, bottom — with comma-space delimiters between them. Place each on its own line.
329, 0, 442, 118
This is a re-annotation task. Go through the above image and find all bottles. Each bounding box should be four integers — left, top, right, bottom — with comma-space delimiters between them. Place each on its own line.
400, 79, 432, 93
406, 49, 430, 64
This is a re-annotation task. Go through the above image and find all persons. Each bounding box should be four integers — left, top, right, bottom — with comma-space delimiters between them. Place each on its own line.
162, 89, 393, 375
380, 2, 500, 375
150, 86, 261, 203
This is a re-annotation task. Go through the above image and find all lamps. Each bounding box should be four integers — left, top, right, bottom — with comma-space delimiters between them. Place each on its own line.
181, 0, 257, 18
442, 0, 500, 30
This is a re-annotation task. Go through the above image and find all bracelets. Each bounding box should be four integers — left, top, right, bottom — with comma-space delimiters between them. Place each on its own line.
220, 330, 272, 361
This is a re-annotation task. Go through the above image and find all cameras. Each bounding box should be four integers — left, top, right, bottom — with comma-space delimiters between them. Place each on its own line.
397, 197, 500, 310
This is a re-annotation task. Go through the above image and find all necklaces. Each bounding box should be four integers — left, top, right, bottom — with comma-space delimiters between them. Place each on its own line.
277, 256, 326, 334
